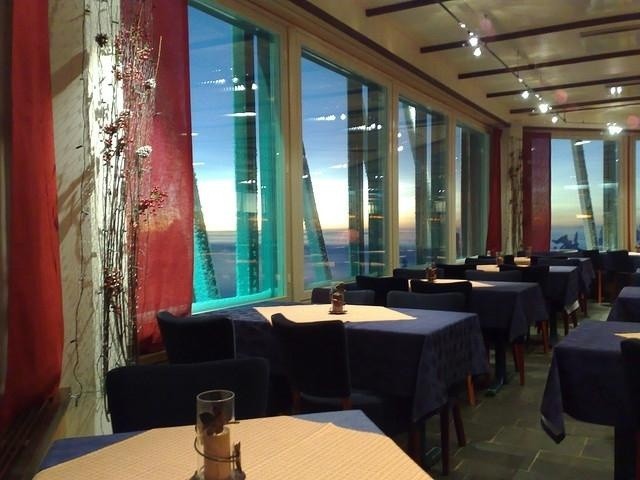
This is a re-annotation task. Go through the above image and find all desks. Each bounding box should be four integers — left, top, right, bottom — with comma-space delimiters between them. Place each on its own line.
553, 266, 638, 475
34, 409, 434, 480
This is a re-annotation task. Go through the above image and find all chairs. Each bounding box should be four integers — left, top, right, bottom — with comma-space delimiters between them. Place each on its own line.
104, 249, 633, 432
